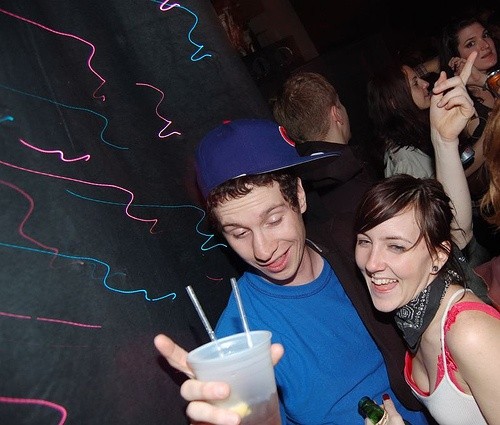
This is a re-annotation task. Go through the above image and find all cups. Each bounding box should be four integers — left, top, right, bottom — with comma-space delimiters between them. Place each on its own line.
187, 330, 282, 425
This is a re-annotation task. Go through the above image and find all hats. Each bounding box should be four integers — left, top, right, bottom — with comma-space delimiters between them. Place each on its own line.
196, 116, 342, 199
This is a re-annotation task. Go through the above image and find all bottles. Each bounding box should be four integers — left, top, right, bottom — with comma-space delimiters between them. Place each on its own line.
358, 396, 412, 425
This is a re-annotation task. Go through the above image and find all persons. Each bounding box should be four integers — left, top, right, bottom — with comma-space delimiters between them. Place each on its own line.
272, 71, 366, 248
368, 58, 435, 179
153, 50, 478, 425
410, 19, 500, 300
355, 173, 500, 425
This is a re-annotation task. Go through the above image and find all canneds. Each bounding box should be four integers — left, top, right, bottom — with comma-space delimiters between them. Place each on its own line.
459, 133, 476, 164
485, 69, 500, 99
